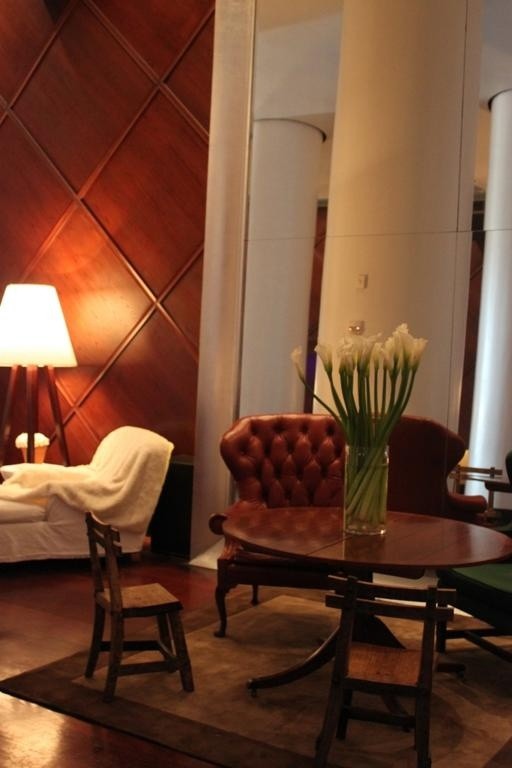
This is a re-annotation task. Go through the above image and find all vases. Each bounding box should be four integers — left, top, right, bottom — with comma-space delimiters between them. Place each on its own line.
341, 441, 390, 538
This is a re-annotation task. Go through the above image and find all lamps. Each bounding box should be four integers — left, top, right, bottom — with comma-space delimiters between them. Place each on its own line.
1, 281, 78, 468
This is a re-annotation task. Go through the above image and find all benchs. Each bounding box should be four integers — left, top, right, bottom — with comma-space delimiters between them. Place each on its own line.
210, 405, 487, 641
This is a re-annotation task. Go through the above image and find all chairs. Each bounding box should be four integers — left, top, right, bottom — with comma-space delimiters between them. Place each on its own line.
435, 450, 510, 665
312, 570, 456, 768
77, 508, 195, 702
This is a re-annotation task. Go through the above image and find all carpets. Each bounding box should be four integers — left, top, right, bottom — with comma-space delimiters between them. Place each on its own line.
0, 584, 510, 767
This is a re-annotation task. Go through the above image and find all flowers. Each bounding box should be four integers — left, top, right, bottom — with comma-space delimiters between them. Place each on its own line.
290, 319, 427, 526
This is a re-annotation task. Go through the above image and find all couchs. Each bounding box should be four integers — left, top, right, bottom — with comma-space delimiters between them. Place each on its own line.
0, 425, 177, 570
